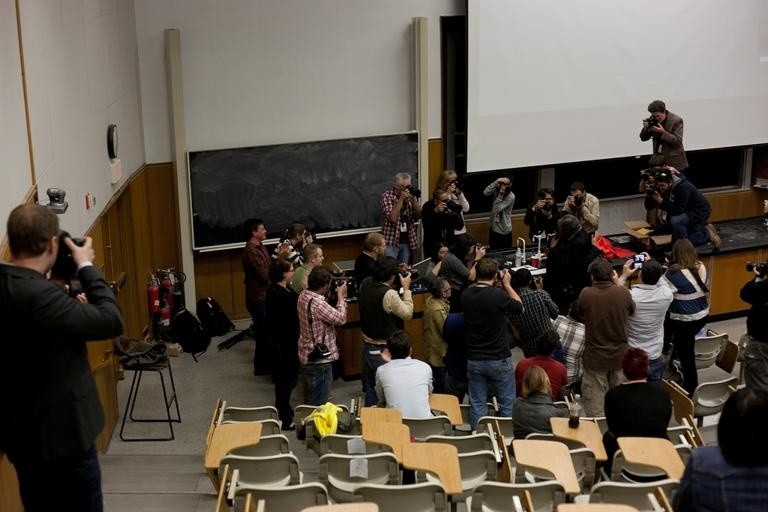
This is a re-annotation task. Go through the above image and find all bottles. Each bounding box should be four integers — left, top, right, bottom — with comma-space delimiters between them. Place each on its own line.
530, 255, 539, 268
568, 393, 580, 428
515, 248, 521, 267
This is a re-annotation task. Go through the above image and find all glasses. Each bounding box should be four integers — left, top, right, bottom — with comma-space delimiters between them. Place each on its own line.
655, 171, 667, 177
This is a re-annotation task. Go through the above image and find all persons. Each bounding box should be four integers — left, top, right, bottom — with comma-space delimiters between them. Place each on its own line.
245, 170, 721, 428
509, 346, 767, 511
374, 331, 435, 486
638, 98, 690, 182
1, 201, 126, 511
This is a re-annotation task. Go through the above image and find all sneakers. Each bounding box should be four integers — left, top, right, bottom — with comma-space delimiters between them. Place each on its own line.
704, 223, 722, 249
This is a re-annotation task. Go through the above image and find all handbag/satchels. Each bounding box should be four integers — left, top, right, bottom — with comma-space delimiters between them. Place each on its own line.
120, 341, 169, 368
196, 296, 233, 336
171, 308, 211, 353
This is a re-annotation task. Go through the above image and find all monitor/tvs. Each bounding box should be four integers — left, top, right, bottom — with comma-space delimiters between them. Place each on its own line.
411, 257, 432, 284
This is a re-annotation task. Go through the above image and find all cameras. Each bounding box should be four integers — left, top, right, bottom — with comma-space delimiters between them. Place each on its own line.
745, 262, 767, 276
302, 230, 316, 250
642, 170, 654, 196
307, 344, 330, 362
500, 182, 512, 195
632, 254, 646, 270
496, 269, 542, 287
474, 241, 490, 254
331, 275, 349, 294
644, 116, 661, 128
542, 193, 584, 211
405, 174, 474, 214
48, 231, 84, 284
396, 269, 407, 282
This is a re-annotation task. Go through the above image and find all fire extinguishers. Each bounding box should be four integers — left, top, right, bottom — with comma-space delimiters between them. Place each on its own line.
144, 264, 186, 326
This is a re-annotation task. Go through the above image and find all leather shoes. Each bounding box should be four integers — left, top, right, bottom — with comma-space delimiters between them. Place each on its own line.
282, 423, 295, 430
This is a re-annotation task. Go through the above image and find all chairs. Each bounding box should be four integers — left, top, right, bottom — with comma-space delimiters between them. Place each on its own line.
115, 336, 181, 443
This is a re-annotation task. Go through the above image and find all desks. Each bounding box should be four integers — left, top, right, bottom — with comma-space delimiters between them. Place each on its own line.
331, 216, 768, 382
